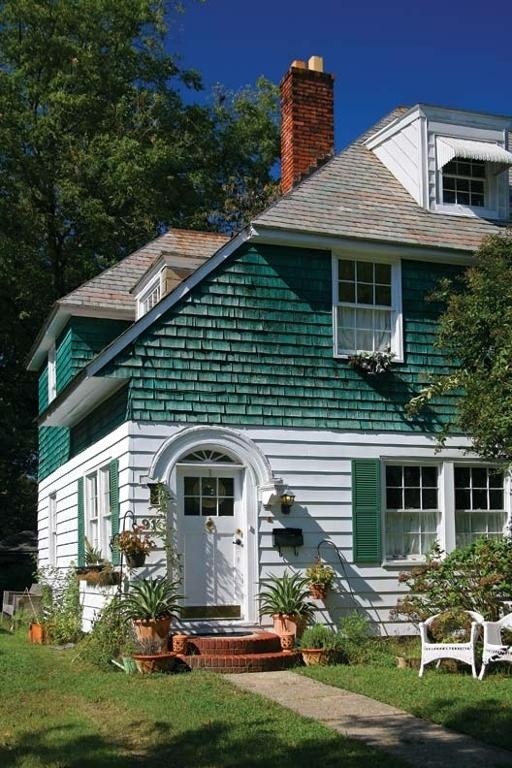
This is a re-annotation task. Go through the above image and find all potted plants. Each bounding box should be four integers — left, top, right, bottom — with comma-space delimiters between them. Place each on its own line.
120, 632, 147, 676
252, 569, 314, 651
110, 574, 187, 650
28, 561, 80, 645
114, 525, 157, 568
391, 636, 421, 670
346, 349, 395, 389
304, 557, 336, 598
78, 547, 105, 573
298, 622, 340, 666
132, 635, 177, 674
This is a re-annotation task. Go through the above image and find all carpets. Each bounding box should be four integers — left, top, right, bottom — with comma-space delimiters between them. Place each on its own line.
191, 631, 254, 637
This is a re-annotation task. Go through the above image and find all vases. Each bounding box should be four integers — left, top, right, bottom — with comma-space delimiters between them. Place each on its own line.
280, 635, 295, 653
173, 635, 188, 657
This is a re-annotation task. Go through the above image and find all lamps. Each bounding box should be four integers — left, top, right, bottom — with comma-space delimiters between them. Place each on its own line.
279, 486, 295, 515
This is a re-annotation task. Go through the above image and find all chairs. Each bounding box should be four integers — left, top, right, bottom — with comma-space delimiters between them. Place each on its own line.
418, 610, 485, 678
477, 612, 512, 678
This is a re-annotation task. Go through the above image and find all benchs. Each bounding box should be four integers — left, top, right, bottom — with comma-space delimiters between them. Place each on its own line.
2, 582, 50, 630
